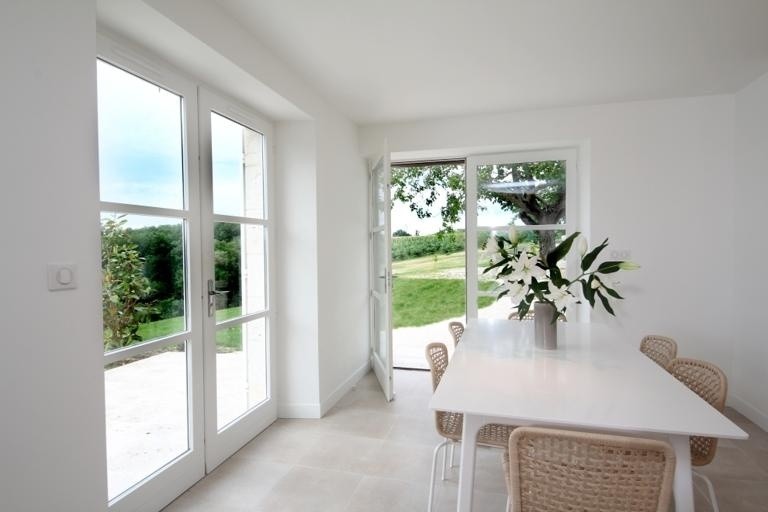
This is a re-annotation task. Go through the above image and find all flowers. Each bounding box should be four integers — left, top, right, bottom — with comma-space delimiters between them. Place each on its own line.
482, 224, 643, 325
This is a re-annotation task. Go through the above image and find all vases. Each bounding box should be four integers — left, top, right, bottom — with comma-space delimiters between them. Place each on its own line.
534, 302, 557, 351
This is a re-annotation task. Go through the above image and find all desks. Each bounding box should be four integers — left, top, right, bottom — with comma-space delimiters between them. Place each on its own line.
430, 318, 749, 512
